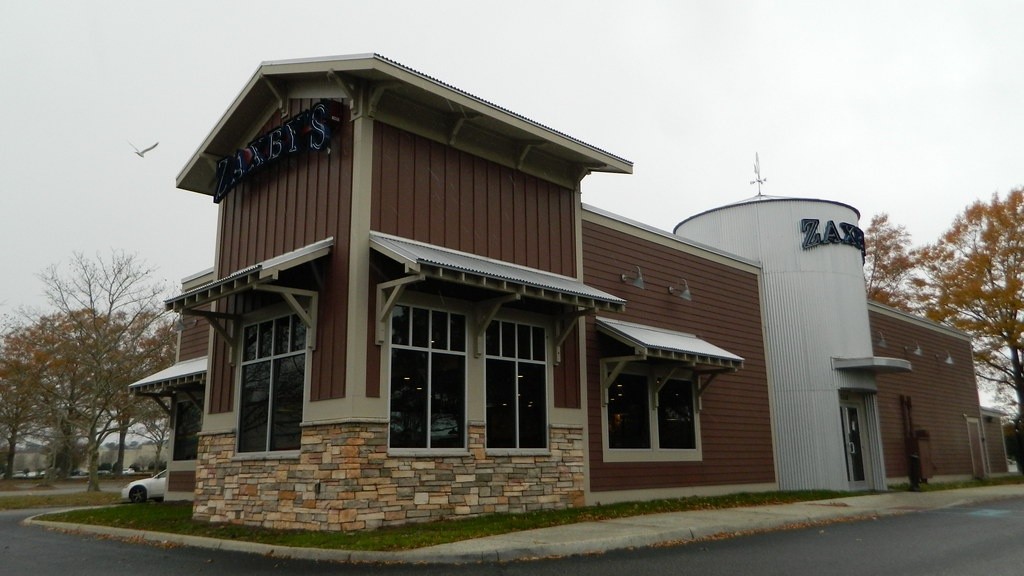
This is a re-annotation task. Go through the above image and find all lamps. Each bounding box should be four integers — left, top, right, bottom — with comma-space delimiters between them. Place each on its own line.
904, 341, 921, 356
175, 312, 198, 329
875, 330, 887, 349
936, 348, 953, 365
621, 265, 644, 289
668, 278, 692, 302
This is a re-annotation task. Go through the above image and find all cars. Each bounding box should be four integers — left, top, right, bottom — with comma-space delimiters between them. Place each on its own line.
96, 471, 110, 475
123, 467, 134, 474
121, 469, 166, 504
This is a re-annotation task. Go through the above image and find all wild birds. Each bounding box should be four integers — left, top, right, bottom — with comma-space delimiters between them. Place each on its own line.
129, 142, 159, 158
